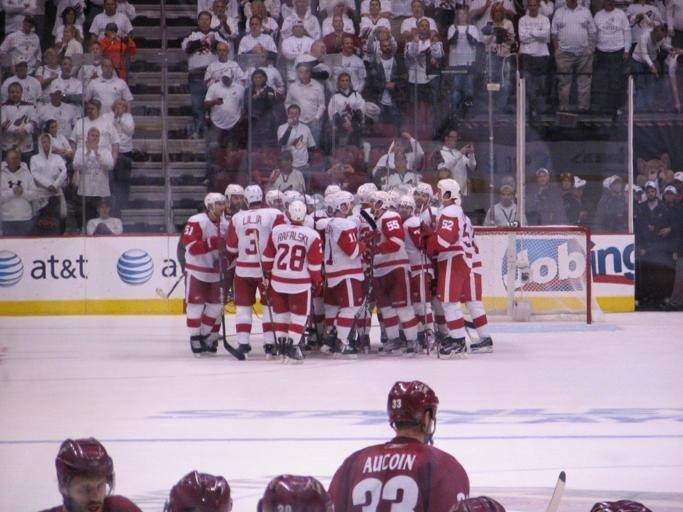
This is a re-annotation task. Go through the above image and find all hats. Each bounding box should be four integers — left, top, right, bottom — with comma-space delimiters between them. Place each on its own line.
609, 175, 622, 186
500, 185, 512, 191
51, 85, 63, 94
560, 172, 572, 180
674, 171, 683, 182
625, 184, 635, 191
536, 167, 548, 175
292, 19, 303, 25
645, 181, 656, 189
602, 178, 611, 187
107, 23, 118, 32
15, 56, 27, 66
635, 186, 643, 193
222, 68, 233, 79
574, 175, 586, 188
663, 185, 676, 194
25, 15, 36, 24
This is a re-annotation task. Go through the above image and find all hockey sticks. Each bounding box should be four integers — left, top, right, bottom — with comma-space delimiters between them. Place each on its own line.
545, 471, 566, 512
420, 217, 441, 356
156, 273, 186, 300
360, 209, 377, 352
217, 245, 245, 362
252, 230, 291, 357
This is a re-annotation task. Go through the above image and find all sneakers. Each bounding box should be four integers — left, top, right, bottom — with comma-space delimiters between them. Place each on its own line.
302, 343, 319, 354
190, 334, 202, 357
438, 343, 469, 359
204, 334, 219, 355
265, 344, 275, 359
378, 347, 402, 356
285, 347, 303, 364
403, 348, 416, 357
320, 344, 333, 355
334, 346, 358, 359
238, 343, 251, 360
470, 336, 494, 353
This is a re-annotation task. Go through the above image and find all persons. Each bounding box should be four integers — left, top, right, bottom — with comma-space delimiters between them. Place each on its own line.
590, 500, 652, 512
1, 0, 137, 236
326, 380, 468, 512
182, 1, 683, 312
38, 437, 142, 512
177, 178, 492, 361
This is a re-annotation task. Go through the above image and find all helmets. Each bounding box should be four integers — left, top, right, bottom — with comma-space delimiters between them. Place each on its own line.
385, 191, 400, 208
335, 191, 354, 205
436, 178, 460, 199
356, 185, 370, 204
225, 183, 244, 200
324, 194, 338, 207
455, 199, 462, 205
305, 194, 312, 205
325, 184, 340, 194
591, 499, 652, 512
288, 200, 307, 222
203, 192, 226, 209
265, 189, 285, 206
286, 190, 300, 204
415, 183, 433, 198
387, 381, 440, 424
400, 195, 415, 208
55, 436, 114, 476
372, 191, 386, 201
243, 184, 264, 204
364, 183, 377, 192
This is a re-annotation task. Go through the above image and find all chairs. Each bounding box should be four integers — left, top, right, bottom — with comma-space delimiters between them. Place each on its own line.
206, 87, 443, 208
